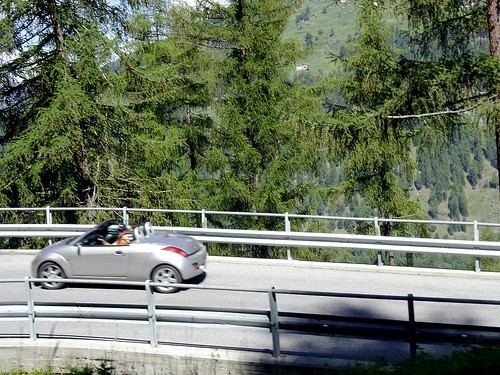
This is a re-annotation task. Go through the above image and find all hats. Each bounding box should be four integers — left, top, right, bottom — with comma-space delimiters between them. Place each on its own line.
118, 225, 126, 232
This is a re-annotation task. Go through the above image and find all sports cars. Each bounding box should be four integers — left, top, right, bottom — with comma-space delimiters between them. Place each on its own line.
31, 219, 208, 293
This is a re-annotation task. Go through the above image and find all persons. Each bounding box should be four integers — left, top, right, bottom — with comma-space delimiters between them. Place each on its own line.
96, 224, 133, 246
128, 223, 139, 233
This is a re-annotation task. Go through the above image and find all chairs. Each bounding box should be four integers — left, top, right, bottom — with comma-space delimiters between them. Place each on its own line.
133, 222, 155, 240
